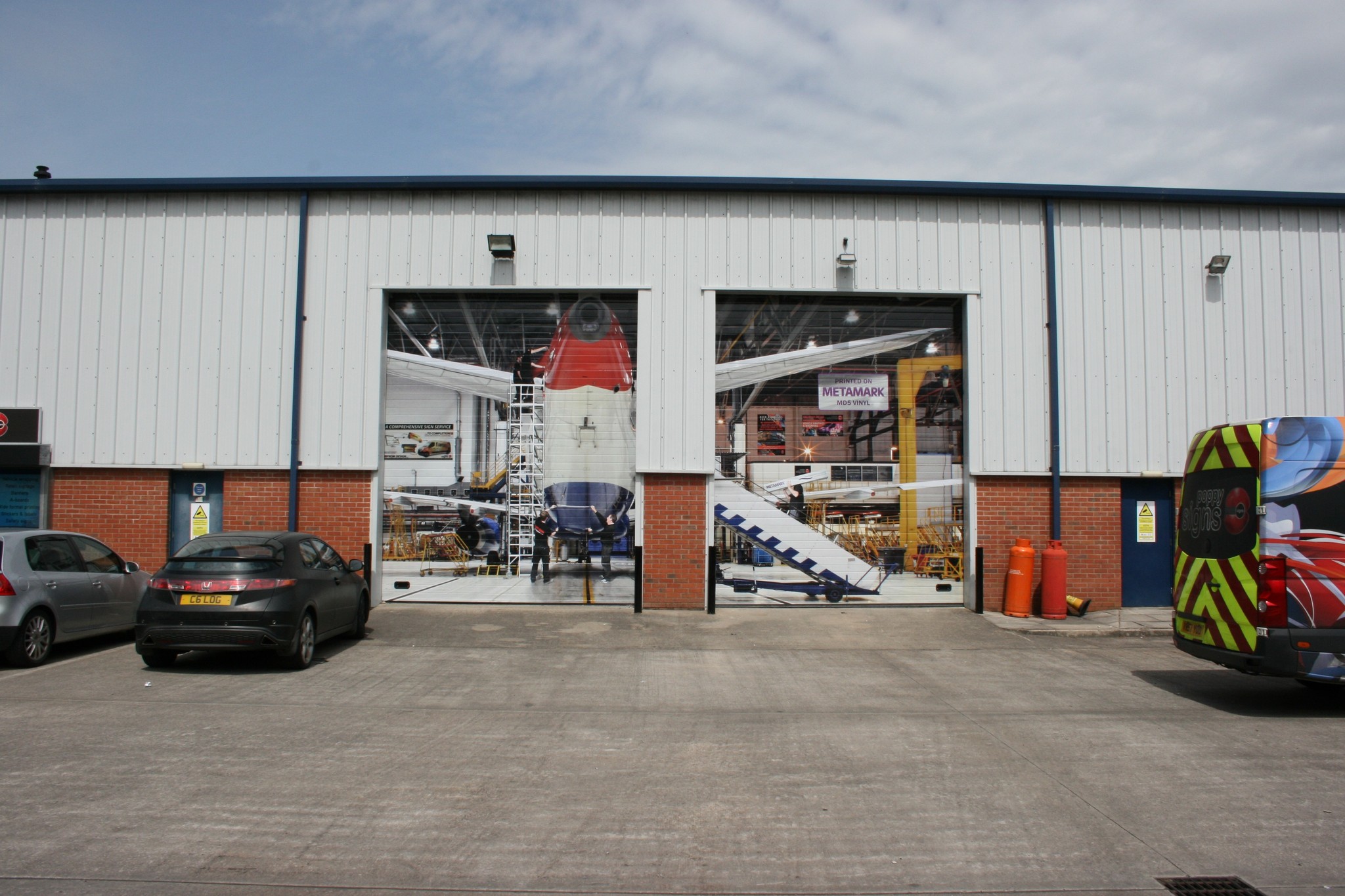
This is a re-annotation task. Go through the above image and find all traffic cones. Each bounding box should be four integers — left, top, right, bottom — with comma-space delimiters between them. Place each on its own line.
1066, 594, 1092, 616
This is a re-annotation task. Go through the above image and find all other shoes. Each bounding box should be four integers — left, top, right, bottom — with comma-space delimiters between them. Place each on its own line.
545, 579, 553, 584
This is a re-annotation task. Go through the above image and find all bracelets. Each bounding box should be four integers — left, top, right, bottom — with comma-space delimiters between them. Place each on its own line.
554, 531, 557, 533
549, 507, 552, 510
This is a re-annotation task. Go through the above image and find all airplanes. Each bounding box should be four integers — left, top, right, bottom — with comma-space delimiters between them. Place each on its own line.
382, 299, 955, 593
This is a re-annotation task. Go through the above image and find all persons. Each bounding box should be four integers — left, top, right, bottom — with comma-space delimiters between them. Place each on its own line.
587, 505, 617, 583
512, 356, 525, 403
522, 346, 548, 404
783, 481, 804, 519
529, 505, 559, 584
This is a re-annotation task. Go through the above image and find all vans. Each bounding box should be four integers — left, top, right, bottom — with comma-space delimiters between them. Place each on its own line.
1171, 415, 1345, 685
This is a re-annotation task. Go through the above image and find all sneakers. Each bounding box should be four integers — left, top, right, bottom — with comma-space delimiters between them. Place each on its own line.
599, 579, 610, 583
599, 575, 604, 578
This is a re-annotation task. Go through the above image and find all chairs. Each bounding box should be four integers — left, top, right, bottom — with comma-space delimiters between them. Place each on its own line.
219, 547, 238, 557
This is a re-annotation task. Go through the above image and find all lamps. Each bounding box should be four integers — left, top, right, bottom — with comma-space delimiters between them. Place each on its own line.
845, 311, 859, 322
546, 303, 559, 316
485, 233, 516, 261
837, 237, 857, 267
1206, 256, 1232, 275
403, 302, 416, 315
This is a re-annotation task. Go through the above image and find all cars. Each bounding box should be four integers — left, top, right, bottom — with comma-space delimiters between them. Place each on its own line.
133, 530, 371, 671
0, 526, 154, 668
